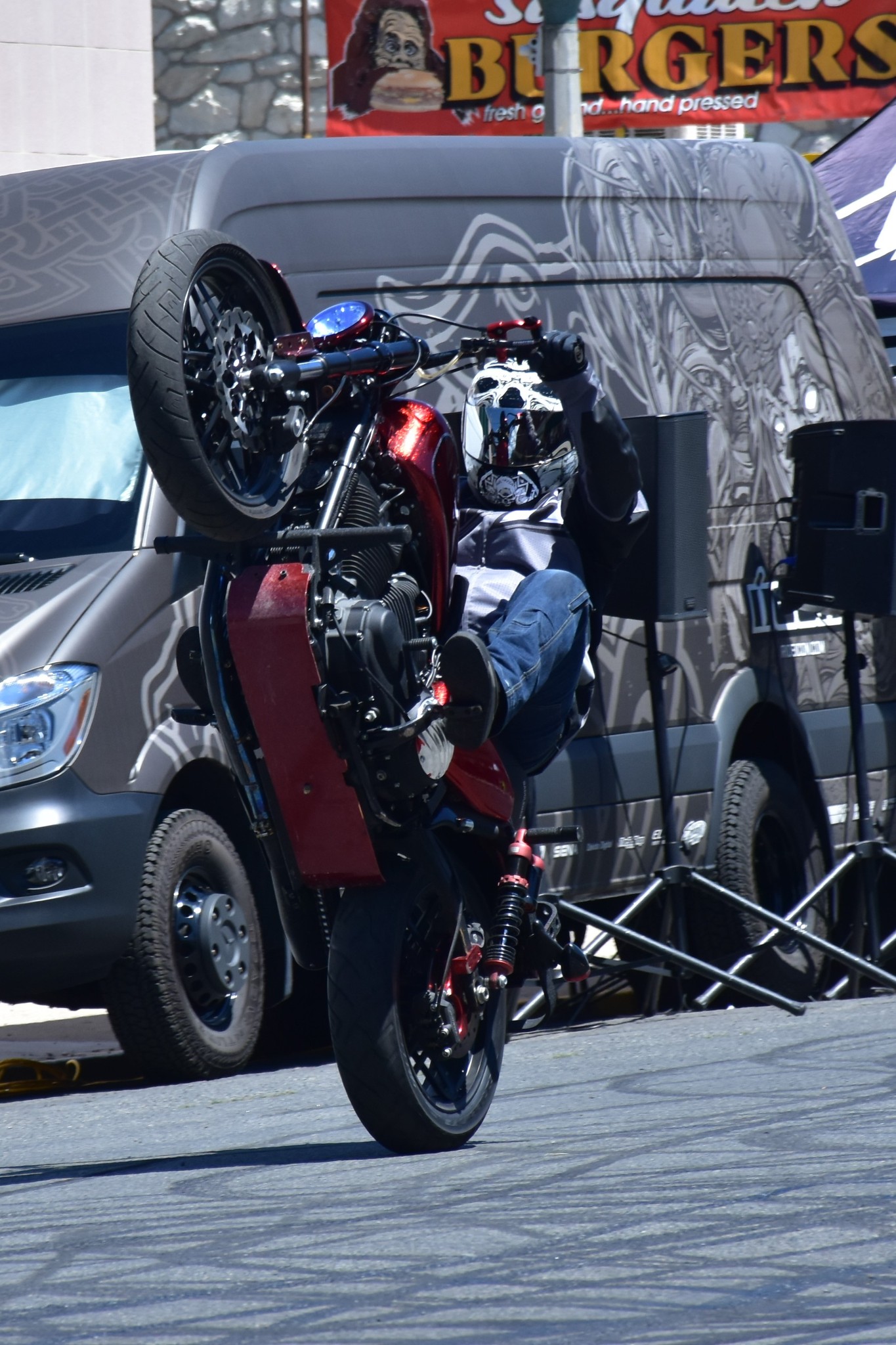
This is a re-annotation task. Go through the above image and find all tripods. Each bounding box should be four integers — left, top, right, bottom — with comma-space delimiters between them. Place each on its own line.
503, 617, 896, 1031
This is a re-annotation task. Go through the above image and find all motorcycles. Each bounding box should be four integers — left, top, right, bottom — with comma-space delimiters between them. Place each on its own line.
127, 226, 592, 1157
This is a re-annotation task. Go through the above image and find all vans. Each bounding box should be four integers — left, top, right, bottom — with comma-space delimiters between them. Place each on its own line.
1, 137, 896, 1081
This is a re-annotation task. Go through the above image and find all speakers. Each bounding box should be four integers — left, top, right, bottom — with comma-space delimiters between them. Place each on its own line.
562, 406, 715, 622
785, 419, 896, 617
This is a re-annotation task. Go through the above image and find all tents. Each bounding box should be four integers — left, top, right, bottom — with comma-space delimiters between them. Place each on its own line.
813, 91, 896, 306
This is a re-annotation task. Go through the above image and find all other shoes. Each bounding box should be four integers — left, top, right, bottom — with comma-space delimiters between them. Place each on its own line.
175, 626, 213, 713
442, 630, 499, 751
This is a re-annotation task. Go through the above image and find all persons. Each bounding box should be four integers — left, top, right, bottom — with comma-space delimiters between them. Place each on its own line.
171, 328, 640, 775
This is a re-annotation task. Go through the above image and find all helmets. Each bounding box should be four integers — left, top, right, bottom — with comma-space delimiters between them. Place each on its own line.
461, 358, 579, 510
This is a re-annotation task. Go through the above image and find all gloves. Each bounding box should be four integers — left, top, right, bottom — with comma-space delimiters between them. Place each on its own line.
529, 330, 589, 383
